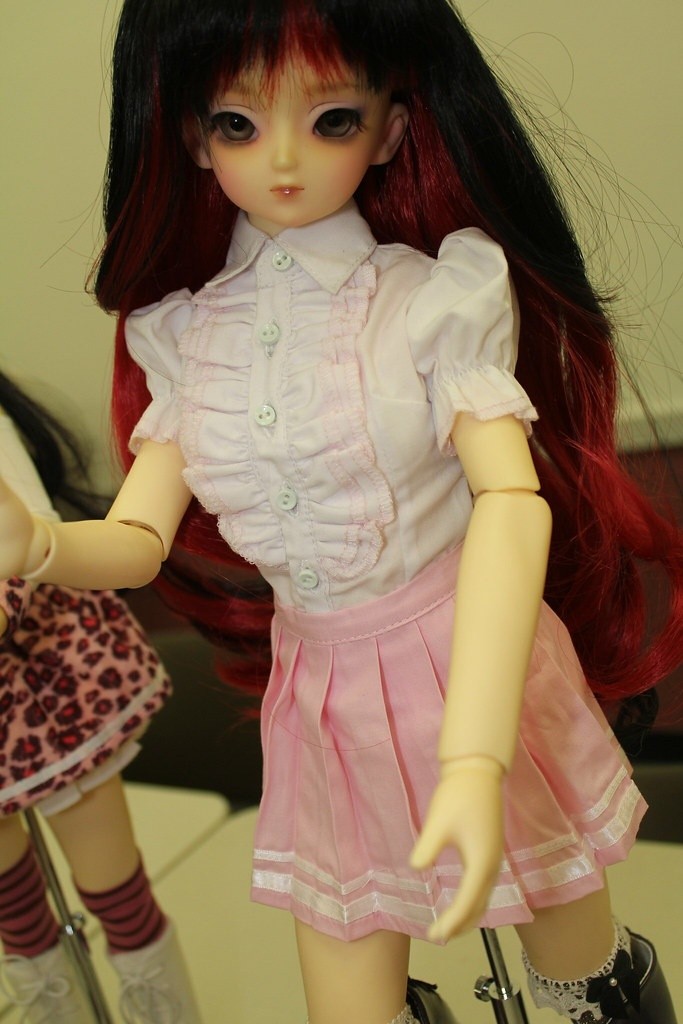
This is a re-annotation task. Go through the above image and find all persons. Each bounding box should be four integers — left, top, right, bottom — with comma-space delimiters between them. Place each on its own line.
0, 1, 683, 1024
0, 366, 197, 1021
